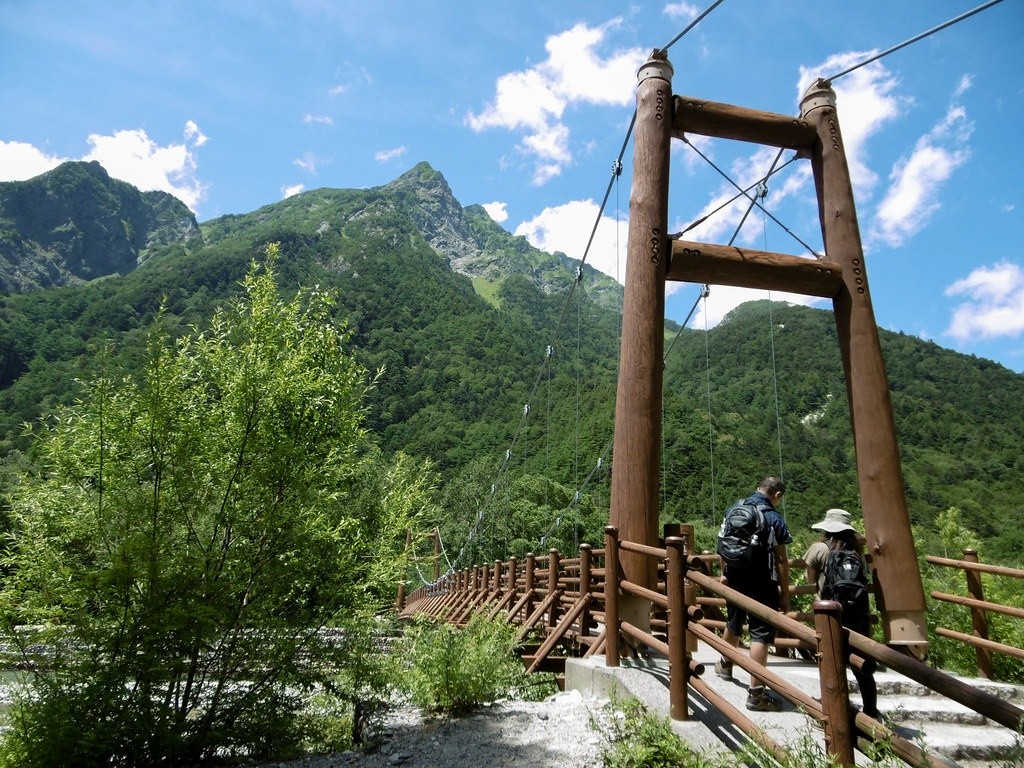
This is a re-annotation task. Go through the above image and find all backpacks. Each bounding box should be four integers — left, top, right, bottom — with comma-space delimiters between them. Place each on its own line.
821, 540, 870, 623
717, 499, 776, 569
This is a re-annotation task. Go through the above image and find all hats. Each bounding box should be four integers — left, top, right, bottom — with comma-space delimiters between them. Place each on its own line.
811, 509, 864, 538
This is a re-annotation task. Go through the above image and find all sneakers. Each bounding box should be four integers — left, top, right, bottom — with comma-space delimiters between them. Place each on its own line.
746, 685, 782, 710
715, 657, 733, 680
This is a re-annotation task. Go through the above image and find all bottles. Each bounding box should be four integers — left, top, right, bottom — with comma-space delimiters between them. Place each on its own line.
751, 535, 760, 545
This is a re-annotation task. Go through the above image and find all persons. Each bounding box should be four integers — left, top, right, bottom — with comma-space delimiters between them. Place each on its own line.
802, 509, 891, 727
715, 476, 793, 712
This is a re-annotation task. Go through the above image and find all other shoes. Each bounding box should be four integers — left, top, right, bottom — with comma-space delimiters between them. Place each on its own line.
873, 710, 887, 722
